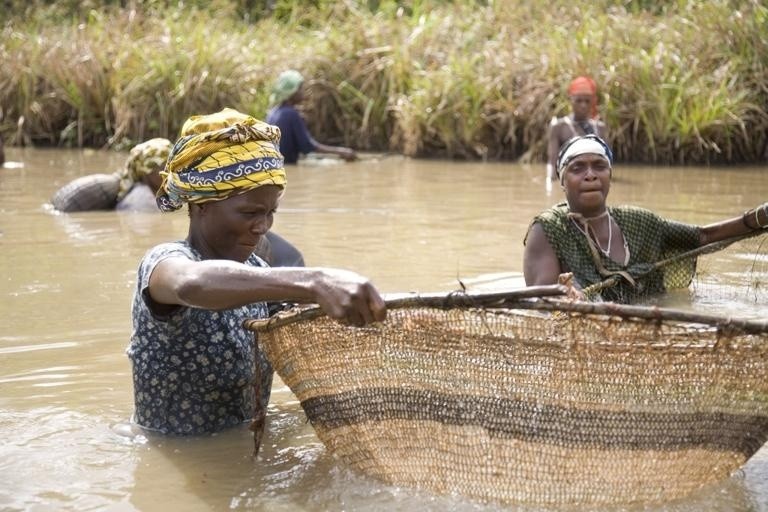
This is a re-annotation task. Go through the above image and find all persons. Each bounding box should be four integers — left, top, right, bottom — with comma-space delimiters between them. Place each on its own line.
266, 70, 357, 164
523, 133, 768, 303
116, 137, 176, 214
127, 108, 387, 435
546, 76, 610, 182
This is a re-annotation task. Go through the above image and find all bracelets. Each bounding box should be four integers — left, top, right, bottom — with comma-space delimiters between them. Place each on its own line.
743, 203, 768, 231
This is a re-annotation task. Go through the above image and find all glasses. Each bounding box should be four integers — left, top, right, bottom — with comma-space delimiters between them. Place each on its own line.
552, 135, 613, 165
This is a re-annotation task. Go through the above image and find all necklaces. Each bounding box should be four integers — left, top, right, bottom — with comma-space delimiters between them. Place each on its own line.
570, 208, 612, 259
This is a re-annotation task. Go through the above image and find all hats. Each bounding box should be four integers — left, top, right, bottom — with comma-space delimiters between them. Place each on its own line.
556, 133, 612, 182
568, 77, 596, 98
